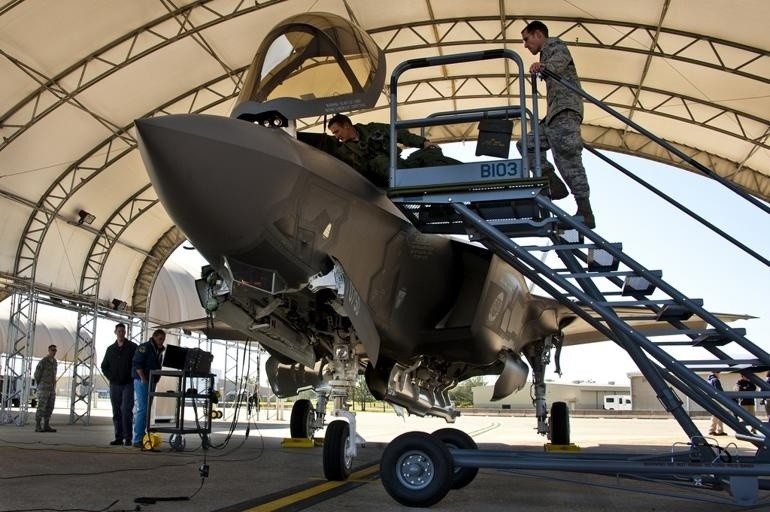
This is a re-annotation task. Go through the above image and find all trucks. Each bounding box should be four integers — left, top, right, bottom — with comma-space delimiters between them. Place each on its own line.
604, 394, 632, 410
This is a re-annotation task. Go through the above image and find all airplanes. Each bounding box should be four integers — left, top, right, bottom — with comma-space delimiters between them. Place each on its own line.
132, 9, 760, 482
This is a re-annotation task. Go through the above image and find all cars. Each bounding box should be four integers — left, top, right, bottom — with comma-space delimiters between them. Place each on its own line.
222, 388, 276, 402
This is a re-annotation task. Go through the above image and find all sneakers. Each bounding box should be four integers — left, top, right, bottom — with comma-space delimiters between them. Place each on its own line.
111, 439, 143, 449
556, 199, 596, 230
542, 171, 568, 200
35, 425, 57, 432
708, 432, 727, 436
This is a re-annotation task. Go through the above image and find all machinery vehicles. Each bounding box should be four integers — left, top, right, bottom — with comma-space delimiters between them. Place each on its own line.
1, 374, 37, 407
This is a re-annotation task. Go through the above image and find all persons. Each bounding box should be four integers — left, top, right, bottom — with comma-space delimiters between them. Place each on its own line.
515, 19, 596, 229
34, 344, 57, 432
132, 330, 166, 448
101, 324, 138, 446
762, 371, 770, 420
734, 374, 757, 437
329, 112, 442, 181
707, 368, 726, 437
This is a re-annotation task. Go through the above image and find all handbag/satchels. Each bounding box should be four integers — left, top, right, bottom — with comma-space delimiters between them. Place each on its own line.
475, 119, 515, 159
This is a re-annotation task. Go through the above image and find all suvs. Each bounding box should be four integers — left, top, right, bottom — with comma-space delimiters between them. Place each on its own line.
94, 389, 109, 402
